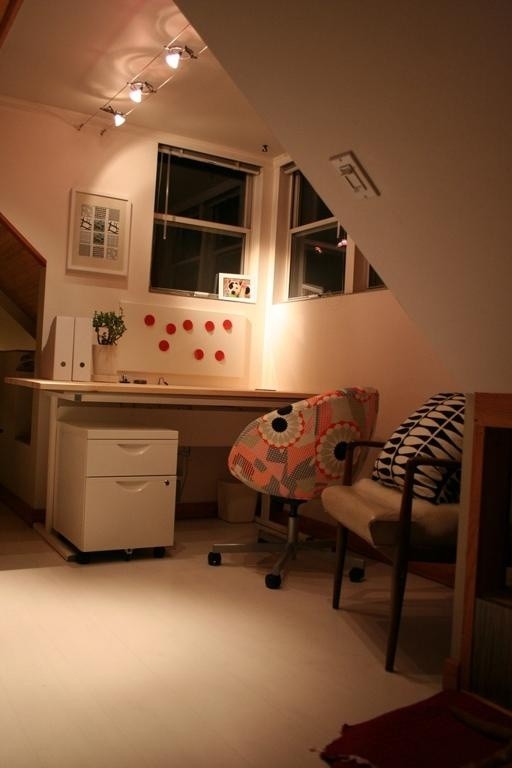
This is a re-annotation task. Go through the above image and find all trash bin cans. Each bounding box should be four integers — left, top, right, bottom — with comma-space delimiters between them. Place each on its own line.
217, 480, 258, 523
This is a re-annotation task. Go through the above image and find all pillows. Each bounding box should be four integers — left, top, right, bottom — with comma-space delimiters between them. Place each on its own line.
370, 389, 467, 507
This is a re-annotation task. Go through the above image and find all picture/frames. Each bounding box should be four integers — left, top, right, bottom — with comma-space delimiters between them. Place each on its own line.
218, 272, 257, 303
66, 187, 132, 278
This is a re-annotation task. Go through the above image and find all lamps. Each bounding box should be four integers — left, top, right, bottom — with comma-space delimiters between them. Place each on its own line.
105, 105, 125, 128
165, 45, 192, 70
128, 80, 155, 103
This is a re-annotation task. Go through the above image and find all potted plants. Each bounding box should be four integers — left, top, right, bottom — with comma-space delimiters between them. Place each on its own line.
90, 305, 129, 382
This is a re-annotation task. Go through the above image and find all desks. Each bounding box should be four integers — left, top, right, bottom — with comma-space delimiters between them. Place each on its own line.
0, 376, 330, 563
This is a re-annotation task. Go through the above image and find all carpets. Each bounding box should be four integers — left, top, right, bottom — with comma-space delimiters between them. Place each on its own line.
316, 690, 511, 767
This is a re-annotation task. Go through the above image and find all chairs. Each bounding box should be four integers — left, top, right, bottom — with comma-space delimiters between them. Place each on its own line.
207, 385, 382, 592
318, 437, 461, 675
